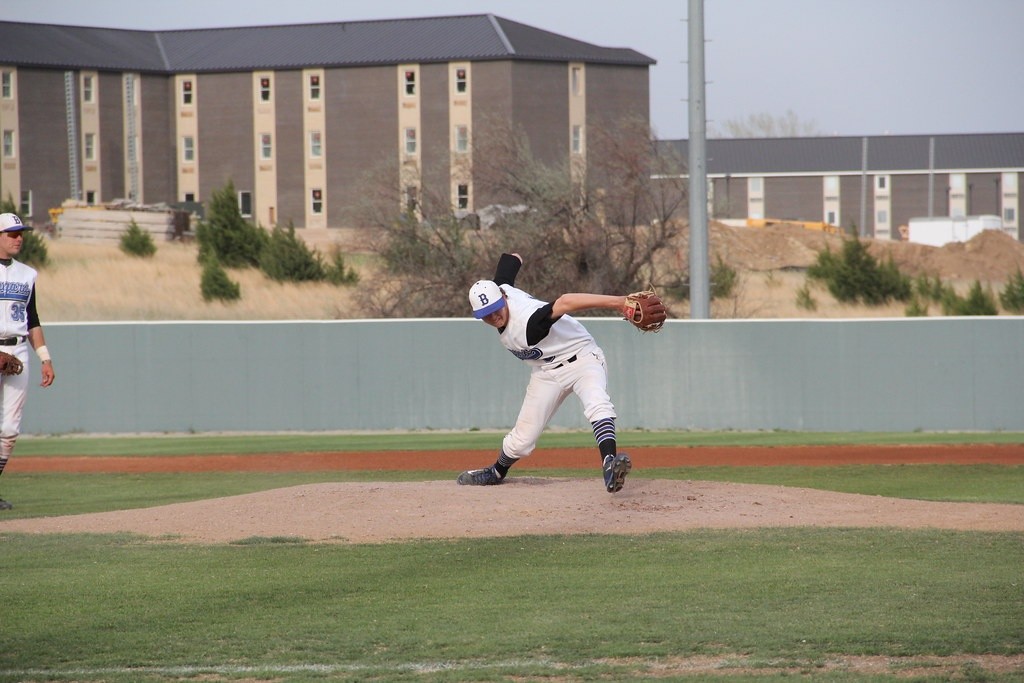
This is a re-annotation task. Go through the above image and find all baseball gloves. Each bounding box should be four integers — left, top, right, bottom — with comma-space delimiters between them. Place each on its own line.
620, 283, 667, 335
0, 351, 24, 376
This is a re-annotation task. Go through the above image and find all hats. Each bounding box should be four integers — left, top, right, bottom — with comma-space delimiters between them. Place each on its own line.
0, 213, 33, 231
469, 280, 506, 319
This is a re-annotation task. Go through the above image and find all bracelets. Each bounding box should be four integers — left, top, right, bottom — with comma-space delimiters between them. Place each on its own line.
36, 346, 51, 362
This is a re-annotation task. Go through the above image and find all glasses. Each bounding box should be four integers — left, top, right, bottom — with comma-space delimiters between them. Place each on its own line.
3, 232, 23, 239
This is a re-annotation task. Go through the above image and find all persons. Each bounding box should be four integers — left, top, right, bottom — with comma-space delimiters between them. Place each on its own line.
458, 252, 667, 493
0, 213, 55, 509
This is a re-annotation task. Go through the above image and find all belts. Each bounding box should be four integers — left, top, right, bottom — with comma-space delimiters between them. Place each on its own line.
553, 354, 577, 369
0, 336, 26, 345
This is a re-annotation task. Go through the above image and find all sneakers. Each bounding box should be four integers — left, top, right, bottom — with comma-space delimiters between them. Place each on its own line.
603, 452, 631, 492
0, 498, 12, 510
457, 466, 506, 486
